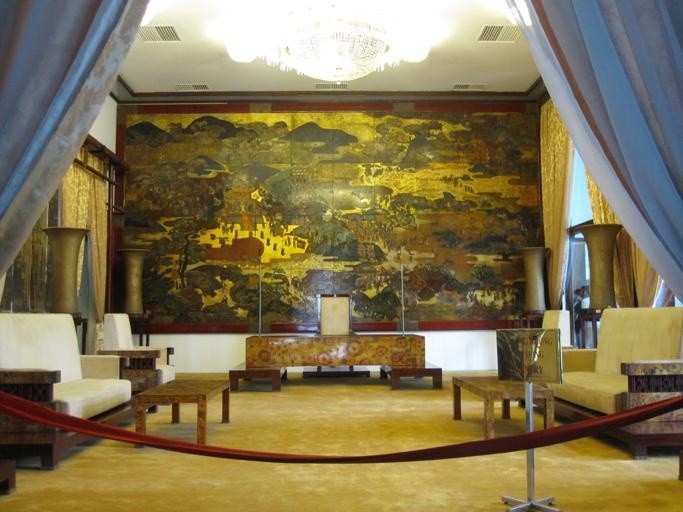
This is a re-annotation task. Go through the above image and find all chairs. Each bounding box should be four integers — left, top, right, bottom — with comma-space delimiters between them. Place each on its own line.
316, 293, 354, 373
95, 313, 176, 413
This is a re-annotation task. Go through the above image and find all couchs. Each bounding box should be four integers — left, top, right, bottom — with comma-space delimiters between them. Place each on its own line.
0, 313, 132, 470
518, 306, 683, 460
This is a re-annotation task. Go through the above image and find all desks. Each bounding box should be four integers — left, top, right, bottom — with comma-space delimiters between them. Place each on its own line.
245, 334, 425, 380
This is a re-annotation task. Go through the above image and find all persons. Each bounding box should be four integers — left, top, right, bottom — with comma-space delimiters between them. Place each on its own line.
573, 286, 587, 348
